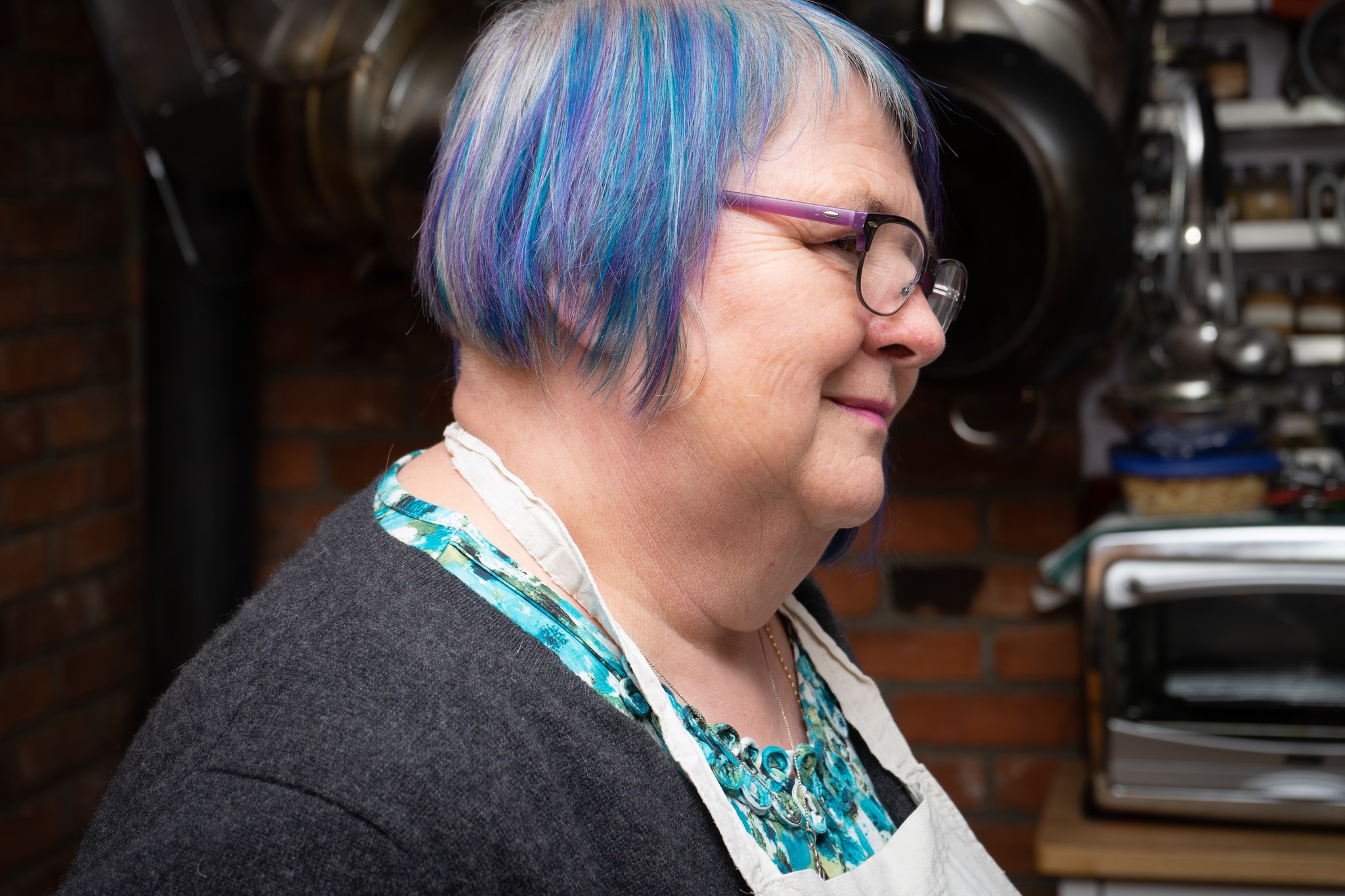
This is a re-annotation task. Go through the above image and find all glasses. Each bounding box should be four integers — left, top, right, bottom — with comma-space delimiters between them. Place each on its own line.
710, 187, 969, 338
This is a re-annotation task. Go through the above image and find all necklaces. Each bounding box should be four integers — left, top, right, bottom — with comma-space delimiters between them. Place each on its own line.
641, 630, 830, 881
764, 622, 804, 717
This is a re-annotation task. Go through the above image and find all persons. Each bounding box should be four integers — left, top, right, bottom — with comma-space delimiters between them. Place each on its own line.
63, 0, 1027, 896
